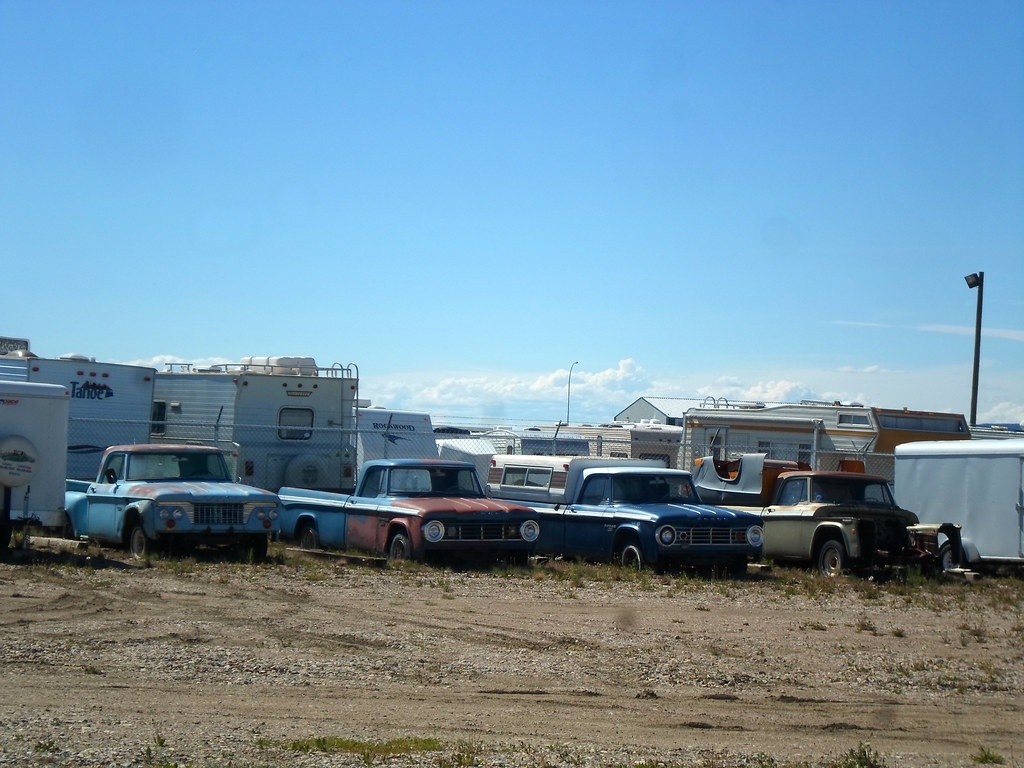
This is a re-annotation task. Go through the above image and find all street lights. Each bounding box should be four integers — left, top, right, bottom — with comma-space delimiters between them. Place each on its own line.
965, 271, 984, 430
566, 362, 579, 424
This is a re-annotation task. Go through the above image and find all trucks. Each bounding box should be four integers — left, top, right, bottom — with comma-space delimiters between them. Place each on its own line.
894, 439, 1024, 578
0, 379, 69, 551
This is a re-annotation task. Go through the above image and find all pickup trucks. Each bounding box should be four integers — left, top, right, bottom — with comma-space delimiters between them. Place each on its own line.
492, 466, 765, 578
278, 458, 543, 570
65, 444, 284, 563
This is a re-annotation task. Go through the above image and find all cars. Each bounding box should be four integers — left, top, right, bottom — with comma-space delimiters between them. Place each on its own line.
711, 470, 967, 581
